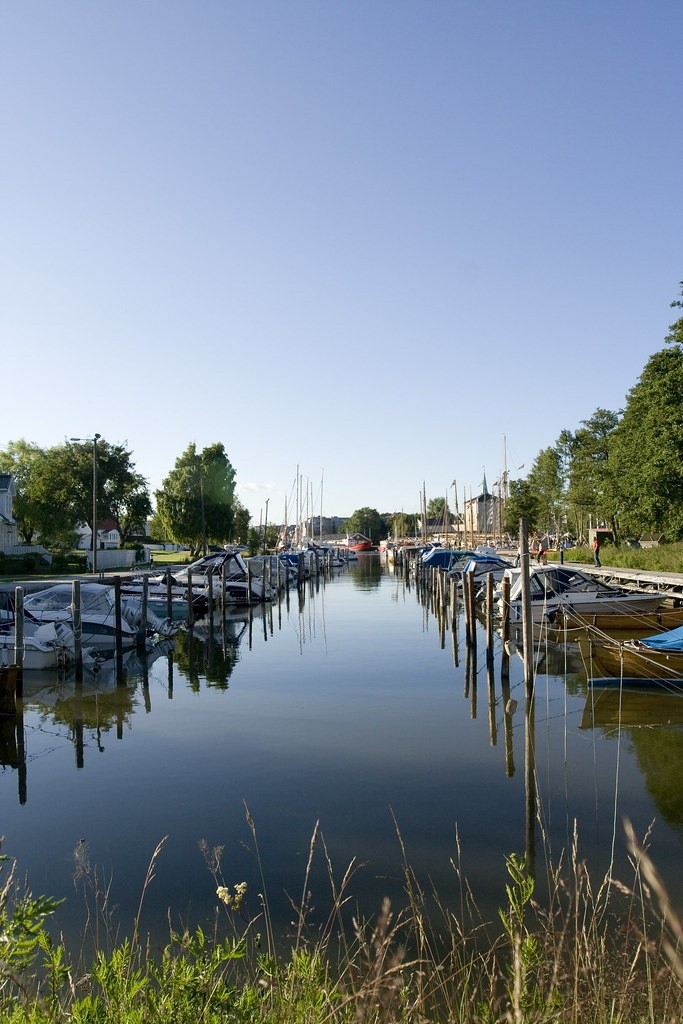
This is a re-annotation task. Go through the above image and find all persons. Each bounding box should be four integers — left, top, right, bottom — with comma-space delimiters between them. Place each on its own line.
593, 536, 601, 567
536, 540, 544, 563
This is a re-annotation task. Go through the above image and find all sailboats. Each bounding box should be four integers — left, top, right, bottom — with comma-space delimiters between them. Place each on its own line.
1, 465, 683, 691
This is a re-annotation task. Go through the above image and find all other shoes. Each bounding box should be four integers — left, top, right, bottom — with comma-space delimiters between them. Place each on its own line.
595, 566, 598, 567
599, 564, 601, 567
